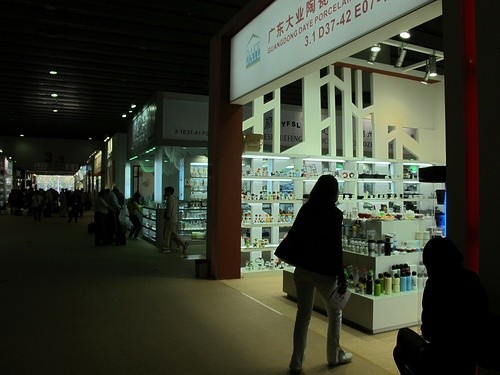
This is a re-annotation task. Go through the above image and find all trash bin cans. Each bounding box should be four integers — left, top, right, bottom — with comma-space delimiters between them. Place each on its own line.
88, 223, 95, 233
195, 259, 209, 278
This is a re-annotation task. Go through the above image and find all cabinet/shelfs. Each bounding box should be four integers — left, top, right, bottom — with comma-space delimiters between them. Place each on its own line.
140, 206, 164, 247
176, 152, 447, 335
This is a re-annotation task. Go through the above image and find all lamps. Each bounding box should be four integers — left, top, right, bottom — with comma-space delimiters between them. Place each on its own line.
365, 29, 411, 70
420, 53, 437, 85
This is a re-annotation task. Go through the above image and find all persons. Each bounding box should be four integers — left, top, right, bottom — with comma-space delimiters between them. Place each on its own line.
288, 174, 352, 375
392, 238, 488, 375
94, 186, 147, 246
8, 184, 92, 223
159, 186, 190, 254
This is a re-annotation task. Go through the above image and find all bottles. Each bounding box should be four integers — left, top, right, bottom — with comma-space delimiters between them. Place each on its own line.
366, 261, 428, 297
385, 238, 391, 256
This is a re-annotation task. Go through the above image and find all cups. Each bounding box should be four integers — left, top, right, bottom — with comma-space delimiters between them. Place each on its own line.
367, 240, 384, 257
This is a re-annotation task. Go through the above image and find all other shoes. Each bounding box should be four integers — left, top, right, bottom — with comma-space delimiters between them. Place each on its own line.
128, 236, 133, 240
183, 240, 190, 252
328, 352, 352, 367
159, 247, 170, 254
134, 236, 141, 240
